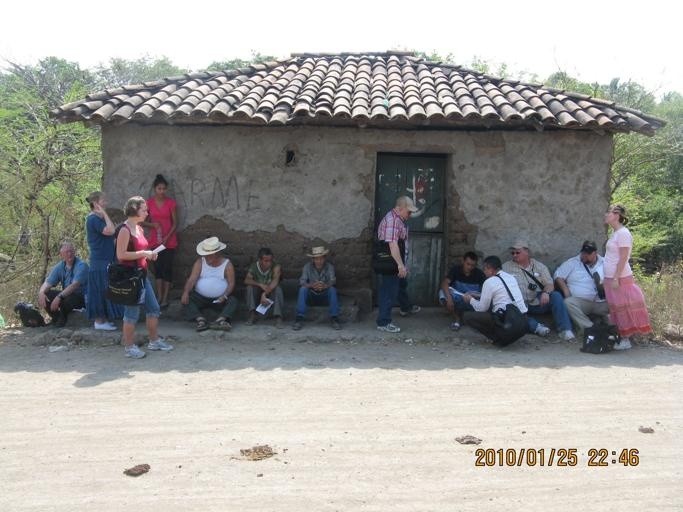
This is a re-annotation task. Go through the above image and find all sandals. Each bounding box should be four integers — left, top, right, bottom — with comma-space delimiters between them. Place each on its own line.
197, 318, 208, 330
209, 319, 232, 330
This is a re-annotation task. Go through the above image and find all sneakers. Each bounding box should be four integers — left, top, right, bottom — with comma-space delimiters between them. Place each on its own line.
400, 304, 421, 316
539, 327, 550, 337
147, 338, 173, 351
293, 319, 304, 330
613, 340, 631, 350
57, 316, 64, 326
331, 319, 341, 329
558, 332, 577, 344
124, 344, 146, 357
377, 322, 400, 332
94, 320, 117, 330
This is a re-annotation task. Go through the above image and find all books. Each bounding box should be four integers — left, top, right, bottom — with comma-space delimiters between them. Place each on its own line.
255, 298, 273, 315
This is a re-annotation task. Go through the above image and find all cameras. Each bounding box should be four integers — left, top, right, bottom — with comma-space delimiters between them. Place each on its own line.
528, 283, 537, 291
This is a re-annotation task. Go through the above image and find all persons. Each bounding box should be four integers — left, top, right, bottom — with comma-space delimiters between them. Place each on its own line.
82, 172, 178, 359
437, 202, 653, 354
372, 196, 422, 334
180, 236, 239, 332
36, 241, 88, 327
241, 248, 284, 330
290, 245, 342, 330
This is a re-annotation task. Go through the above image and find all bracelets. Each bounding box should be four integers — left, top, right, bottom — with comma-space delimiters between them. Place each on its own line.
57, 292, 64, 299
223, 294, 227, 299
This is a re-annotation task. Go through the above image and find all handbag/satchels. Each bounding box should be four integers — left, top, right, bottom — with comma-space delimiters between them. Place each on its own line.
504, 304, 526, 336
373, 238, 405, 273
14, 303, 42, 327
583, 326, 617, 354
106, 262, 145, 305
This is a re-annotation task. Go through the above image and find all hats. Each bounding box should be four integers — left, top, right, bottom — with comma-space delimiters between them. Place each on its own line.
396, 196, 419, 212
580, 240, 597, 254
196, 236, 226, 256
307, 246, 329, 258
509, 242, 530, 250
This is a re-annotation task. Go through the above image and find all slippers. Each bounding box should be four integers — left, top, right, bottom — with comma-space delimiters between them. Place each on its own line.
451, 320, 460, 331
